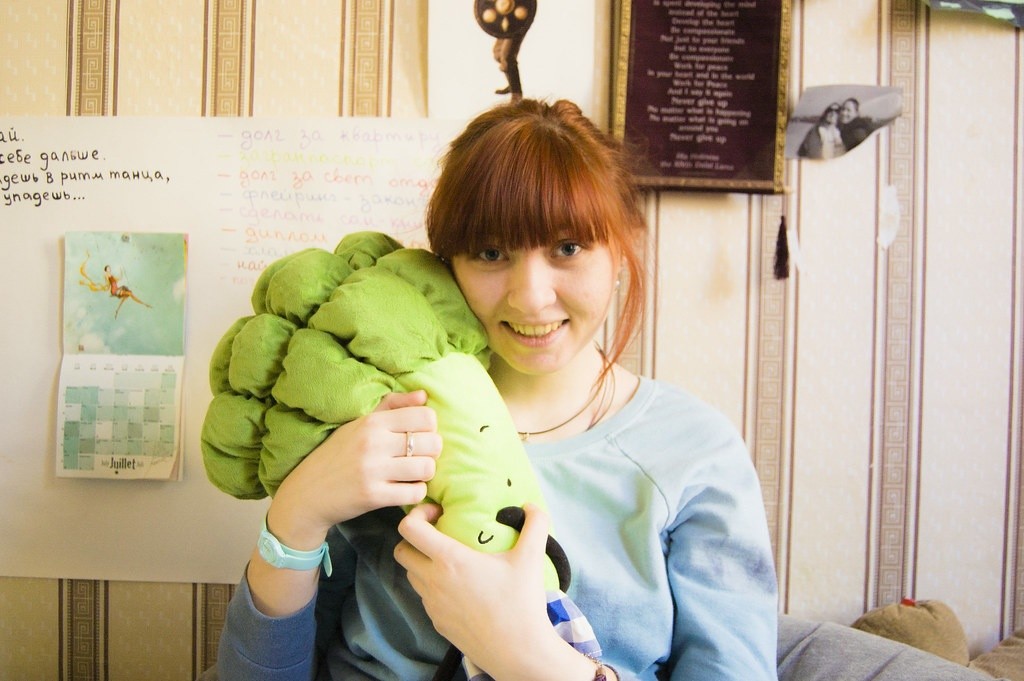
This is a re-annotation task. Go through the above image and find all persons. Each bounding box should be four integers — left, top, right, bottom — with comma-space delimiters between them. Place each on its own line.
490, 0, 539, 105
839, 98, 880, 151
796, 101, 848, 161
203, 94, 786, 680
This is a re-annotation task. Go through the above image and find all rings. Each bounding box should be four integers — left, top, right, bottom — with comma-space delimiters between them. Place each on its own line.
405, 431, 416, 458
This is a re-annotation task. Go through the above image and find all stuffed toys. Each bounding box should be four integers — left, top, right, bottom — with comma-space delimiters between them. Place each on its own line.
201, 222, 608, 681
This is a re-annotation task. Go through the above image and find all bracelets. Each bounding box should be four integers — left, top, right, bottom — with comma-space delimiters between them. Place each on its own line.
586, 658, 608, 681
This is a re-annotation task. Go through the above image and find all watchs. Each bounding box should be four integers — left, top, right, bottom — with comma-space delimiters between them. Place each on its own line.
256, 507, 334, 580
